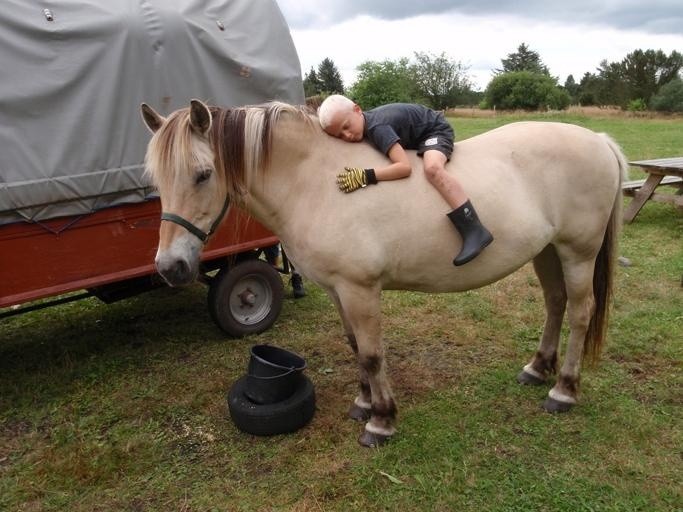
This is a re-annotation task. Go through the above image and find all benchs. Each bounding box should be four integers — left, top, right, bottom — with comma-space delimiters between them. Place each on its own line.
621, 175, 683, 190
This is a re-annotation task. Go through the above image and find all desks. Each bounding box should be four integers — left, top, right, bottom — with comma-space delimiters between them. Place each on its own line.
620, 157, 683, 224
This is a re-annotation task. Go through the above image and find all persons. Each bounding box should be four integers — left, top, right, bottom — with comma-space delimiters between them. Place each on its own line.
315, 93, 493, 266
263, 243, 305, 298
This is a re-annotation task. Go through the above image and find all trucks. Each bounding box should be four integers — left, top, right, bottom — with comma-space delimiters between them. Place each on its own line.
0, 1, 308, 343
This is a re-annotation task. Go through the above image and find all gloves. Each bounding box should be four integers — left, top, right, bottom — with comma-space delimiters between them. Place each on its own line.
336, 166, 377, 194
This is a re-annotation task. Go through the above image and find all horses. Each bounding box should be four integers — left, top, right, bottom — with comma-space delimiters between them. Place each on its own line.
139, 99, 629, 447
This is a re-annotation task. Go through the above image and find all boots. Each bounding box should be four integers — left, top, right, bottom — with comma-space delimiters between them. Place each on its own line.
446, 199, 493, 266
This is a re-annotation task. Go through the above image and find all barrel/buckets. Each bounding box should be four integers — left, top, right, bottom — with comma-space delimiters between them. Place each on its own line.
243, 343, 308, 406
